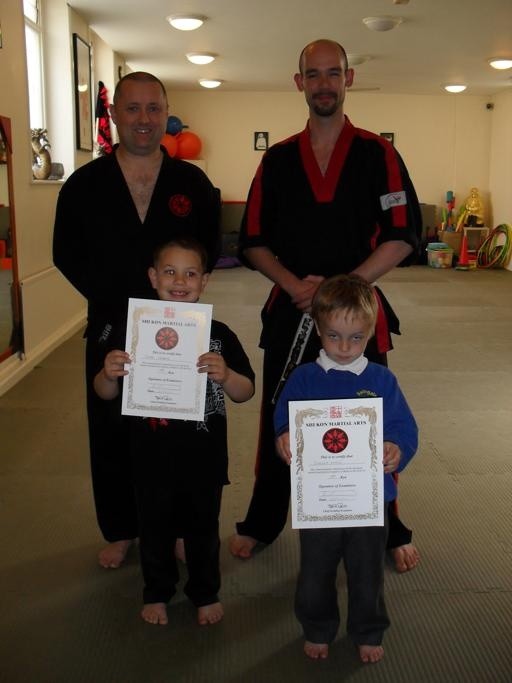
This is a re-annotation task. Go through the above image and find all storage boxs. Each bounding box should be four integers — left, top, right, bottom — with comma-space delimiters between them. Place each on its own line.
439, 231, 463, 252
426, 246, 453, 269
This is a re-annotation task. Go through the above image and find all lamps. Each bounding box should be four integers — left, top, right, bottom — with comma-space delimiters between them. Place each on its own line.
488, 56, 512, 70
199, 78, 222, 88
346, 55, 372, 67
364, 17, 403, 32
168, 14, 207, 30
184, 51, 219, 65
444, 83, 467, 94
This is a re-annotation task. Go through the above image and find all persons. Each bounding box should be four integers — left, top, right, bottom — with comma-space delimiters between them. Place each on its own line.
229, 39, 426, 574
53, 72, 223, 568
94, 242, 254, 629
271, 272, 418, 664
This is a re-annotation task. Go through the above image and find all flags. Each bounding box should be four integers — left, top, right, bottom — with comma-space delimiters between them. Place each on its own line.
96, 82, 114, 152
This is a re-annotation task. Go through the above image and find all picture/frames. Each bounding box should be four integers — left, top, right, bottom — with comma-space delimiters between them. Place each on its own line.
73, 32, 94, 153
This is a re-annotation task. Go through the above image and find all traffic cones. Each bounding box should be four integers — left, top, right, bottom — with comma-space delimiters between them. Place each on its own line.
455, 236, 473, 271
